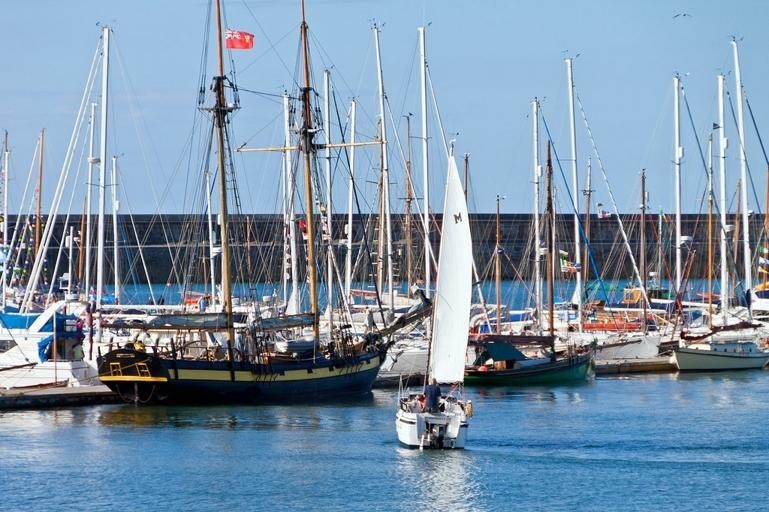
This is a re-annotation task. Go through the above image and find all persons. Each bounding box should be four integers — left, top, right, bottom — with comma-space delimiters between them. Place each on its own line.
149, 295, 164, 305
69, 342, 85, 362
198, 295, 209, 312
423, 378, 442, 412
363, 308, 373, 335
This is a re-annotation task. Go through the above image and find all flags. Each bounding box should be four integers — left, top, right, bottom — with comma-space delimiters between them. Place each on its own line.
225, 30, 254, 49
599, 210, 611, 219
560, 258, 582, 272
